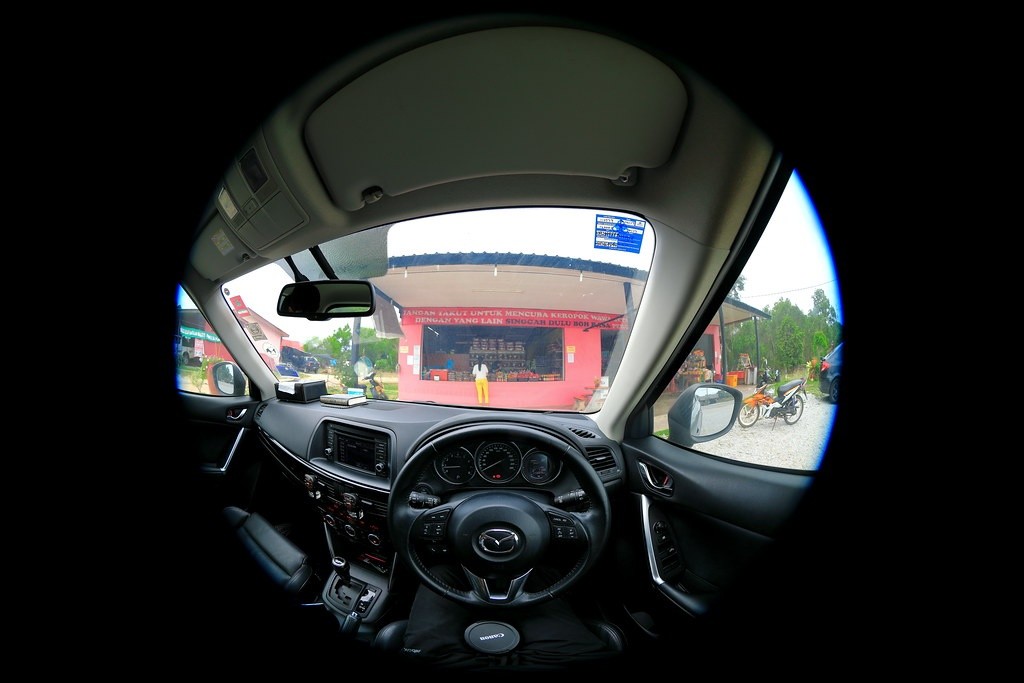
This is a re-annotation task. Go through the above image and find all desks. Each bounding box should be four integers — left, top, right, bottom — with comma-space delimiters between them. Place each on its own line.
585, 386, 609, 399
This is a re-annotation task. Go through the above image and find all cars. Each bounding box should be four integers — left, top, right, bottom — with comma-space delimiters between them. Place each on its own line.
314, 354, 337, 368
817, 342, 843, 403
293, 356, 321, 374
175, 335, 204, 366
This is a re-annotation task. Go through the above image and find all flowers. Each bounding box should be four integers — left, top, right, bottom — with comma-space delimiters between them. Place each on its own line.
594, 378, 601, 383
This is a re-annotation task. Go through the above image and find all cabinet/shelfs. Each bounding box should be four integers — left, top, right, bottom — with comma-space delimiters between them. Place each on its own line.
498, 351, 525, 370
469, 350, 498, 369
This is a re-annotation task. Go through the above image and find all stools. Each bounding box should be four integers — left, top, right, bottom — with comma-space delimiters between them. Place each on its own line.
573, 395, 587, 412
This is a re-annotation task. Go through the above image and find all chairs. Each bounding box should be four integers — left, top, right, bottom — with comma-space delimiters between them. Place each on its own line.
227, 505, 312, 596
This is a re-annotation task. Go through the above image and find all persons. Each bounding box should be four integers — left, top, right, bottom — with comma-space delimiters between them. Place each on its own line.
472, 356, 490, 406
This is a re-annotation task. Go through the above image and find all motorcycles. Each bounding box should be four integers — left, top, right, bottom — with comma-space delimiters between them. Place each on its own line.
738, 369, 804, 432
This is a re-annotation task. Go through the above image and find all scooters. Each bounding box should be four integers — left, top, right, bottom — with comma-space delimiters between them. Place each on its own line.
342, 372, 389, 400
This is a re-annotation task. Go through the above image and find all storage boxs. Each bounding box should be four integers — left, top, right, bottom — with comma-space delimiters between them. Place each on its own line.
726, 374, 738, 388
430, 369, 450, 381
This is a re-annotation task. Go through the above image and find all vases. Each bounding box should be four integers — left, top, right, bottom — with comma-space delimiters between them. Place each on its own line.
596, 383, 599, 387
